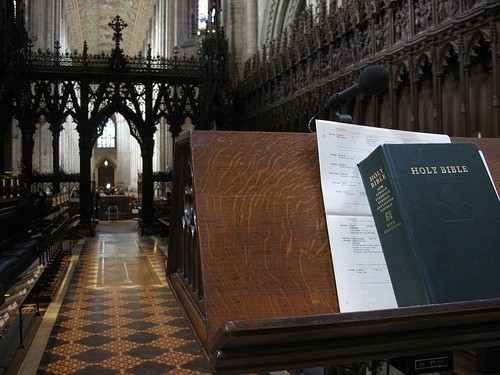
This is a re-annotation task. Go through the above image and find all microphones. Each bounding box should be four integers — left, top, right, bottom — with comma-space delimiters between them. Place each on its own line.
327, 65, 390, 111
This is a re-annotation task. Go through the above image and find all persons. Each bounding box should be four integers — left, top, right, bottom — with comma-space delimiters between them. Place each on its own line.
88, 185, 137, 212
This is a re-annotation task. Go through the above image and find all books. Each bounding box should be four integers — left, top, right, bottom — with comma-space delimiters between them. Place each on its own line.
358, 143, 500, 304
